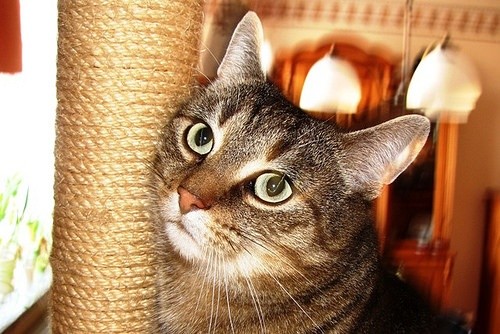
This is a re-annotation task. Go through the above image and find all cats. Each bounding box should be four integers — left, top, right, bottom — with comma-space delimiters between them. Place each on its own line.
139, 10, 476, 334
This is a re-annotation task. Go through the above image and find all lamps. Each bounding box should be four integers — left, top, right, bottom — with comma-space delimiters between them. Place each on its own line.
406, 31, 483, 124
299, 42, 363, 114
